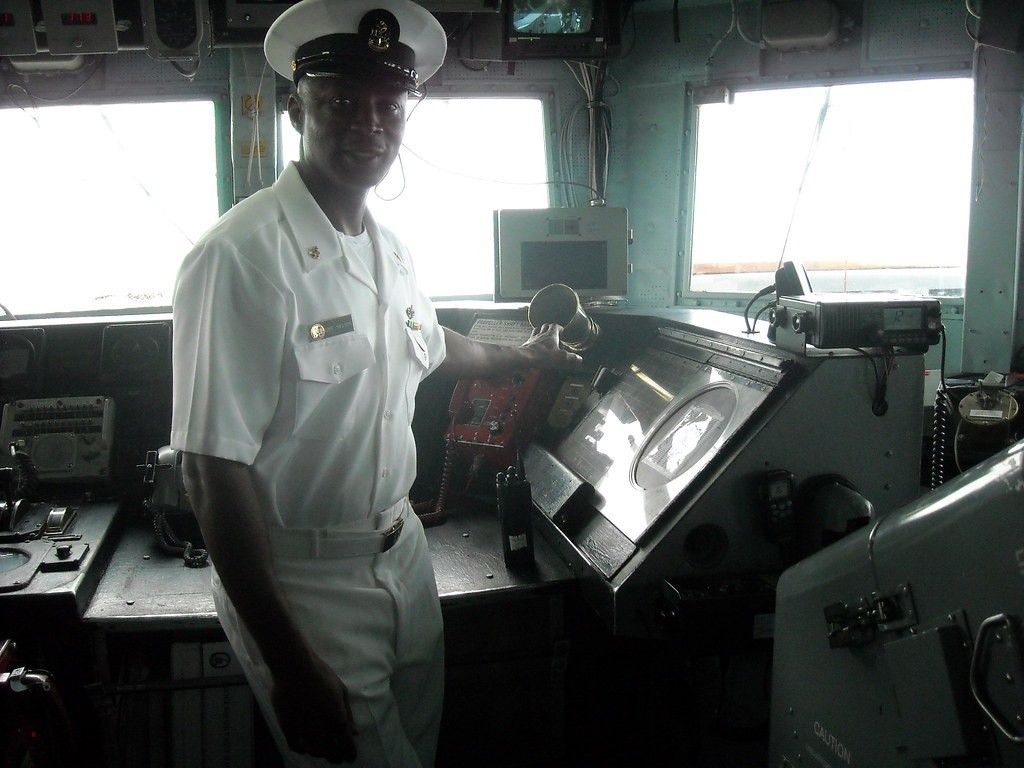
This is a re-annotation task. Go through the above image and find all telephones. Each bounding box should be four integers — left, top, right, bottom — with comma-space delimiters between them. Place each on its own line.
443, 367, 554, 466
148, 443, 193, 514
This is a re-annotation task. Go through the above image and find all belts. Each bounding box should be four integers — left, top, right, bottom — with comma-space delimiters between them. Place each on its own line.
257, 503, 408, 561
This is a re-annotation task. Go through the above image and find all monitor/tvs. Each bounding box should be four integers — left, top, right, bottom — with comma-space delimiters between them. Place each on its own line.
500, 0, 610, 60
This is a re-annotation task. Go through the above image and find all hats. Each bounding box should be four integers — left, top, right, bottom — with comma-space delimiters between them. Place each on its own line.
263, 0, 447, 97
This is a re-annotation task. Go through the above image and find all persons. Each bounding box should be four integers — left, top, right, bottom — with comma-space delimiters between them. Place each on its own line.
169, 0, 584, 768
